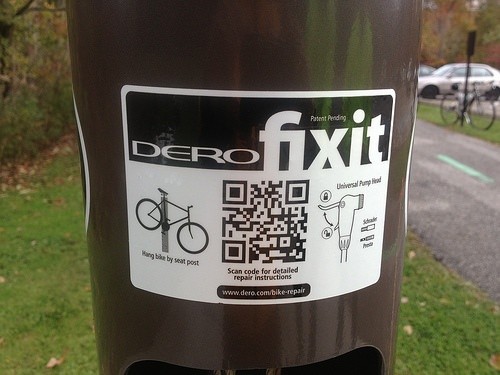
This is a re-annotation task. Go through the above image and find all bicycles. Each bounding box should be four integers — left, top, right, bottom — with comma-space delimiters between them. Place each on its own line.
440, 84, 495, 132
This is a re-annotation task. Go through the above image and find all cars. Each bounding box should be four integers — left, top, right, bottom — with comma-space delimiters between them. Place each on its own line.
416, 63, 436, 77
416, 63, 500, 101
